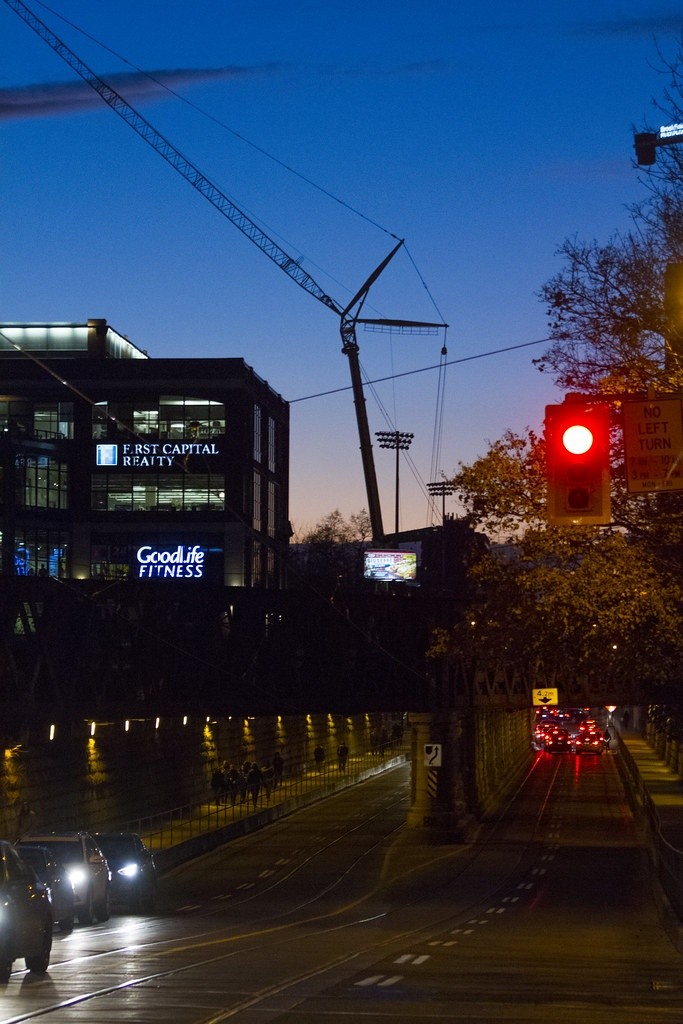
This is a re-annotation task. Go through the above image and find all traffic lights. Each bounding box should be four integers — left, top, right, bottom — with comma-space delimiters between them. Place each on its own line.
543, 403, 611, 528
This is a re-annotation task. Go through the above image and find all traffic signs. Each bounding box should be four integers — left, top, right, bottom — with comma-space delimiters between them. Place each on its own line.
532, 687, 559, 706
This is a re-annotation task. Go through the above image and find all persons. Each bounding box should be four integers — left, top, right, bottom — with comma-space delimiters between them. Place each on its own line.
603, 729, 611, 749
623, 710, 629, 728
21, 564, 48, 577
211, 750, 284, 807
370, 722, 404, 757
314, 744, 326, 773
337, 741, 348, 772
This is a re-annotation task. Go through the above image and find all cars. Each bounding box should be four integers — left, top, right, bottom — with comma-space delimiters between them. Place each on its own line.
0, 838, 54, 983
533, 706, 611, 756
9, 827, 161, 932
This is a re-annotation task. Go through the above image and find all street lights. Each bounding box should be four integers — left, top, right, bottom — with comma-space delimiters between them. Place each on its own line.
375, 430, 416, 549
425, 480, 459, 597
